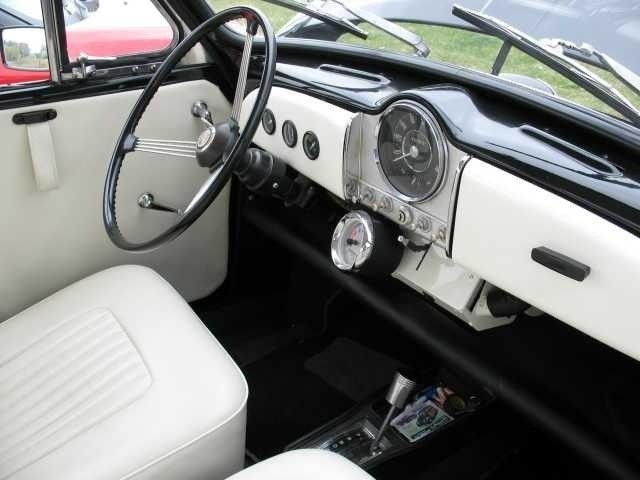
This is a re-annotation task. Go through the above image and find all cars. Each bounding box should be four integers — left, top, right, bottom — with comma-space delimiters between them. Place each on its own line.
2, 0, 173, 85
